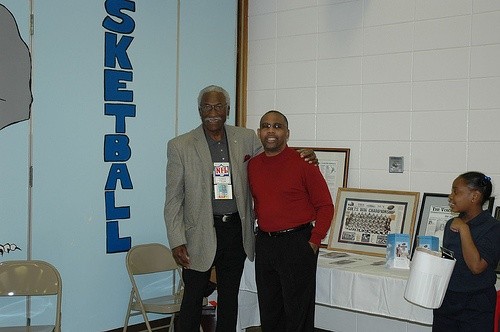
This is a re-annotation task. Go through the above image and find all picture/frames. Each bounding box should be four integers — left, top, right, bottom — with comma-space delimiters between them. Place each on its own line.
291, 147, 495, 261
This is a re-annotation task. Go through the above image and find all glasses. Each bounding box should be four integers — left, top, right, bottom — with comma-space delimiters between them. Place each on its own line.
260, 122, 288, 129
199, 104, 228, 112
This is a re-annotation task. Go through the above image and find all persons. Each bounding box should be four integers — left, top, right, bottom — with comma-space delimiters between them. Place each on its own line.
432, 172, 500, 332
163, 86, 319, 332
247, 111, 334, 332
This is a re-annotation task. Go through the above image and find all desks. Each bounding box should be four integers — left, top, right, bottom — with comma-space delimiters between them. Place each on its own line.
236, 248, 433, 332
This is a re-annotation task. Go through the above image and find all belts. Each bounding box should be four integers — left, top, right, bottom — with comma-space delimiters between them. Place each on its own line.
213, 212, 241, 223
256, 222, 312, 238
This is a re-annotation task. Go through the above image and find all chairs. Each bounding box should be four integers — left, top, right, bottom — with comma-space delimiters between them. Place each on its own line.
123, 244, 208, 332
0, 260, 61, 332
